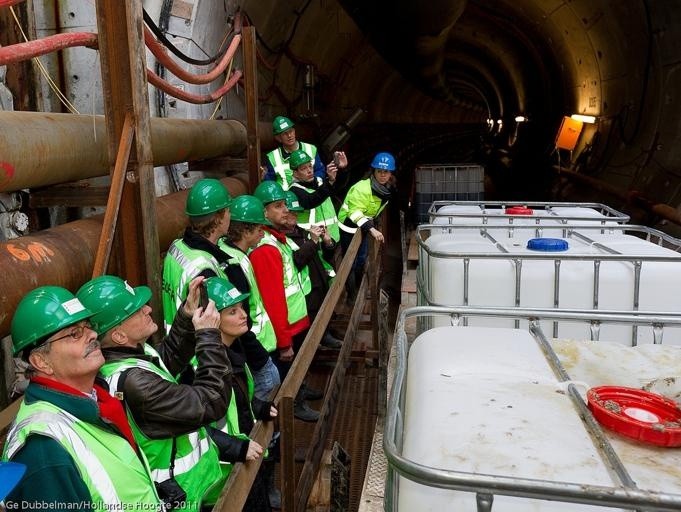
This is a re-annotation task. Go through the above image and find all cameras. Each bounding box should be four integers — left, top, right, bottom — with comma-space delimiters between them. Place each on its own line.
319, 226, 325, 233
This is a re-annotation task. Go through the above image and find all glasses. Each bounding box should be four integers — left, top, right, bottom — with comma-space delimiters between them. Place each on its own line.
50, 321, 91, 345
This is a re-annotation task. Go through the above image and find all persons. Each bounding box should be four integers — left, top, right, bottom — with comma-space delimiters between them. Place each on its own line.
0, 115, 349, 512
336, 152, 396, 308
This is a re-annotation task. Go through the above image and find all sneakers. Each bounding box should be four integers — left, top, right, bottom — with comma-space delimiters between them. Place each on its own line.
293, 402, 320, 422
305, 389, 323, 400
321, 335, 342, 348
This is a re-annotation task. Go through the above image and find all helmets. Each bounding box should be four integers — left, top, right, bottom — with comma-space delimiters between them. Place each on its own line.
10, 284, 99, 352
371, 153, 395, 170
200, 277, 253, 314
228, 181, 303, 225
272, 116, 294, 134
184, 179, 233, 216
74, 275, 152, 337
290, 150, 313, 169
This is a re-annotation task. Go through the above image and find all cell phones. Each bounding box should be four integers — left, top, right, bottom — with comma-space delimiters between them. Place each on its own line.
334, 153, 340, 165
199, 282, 209, 311
